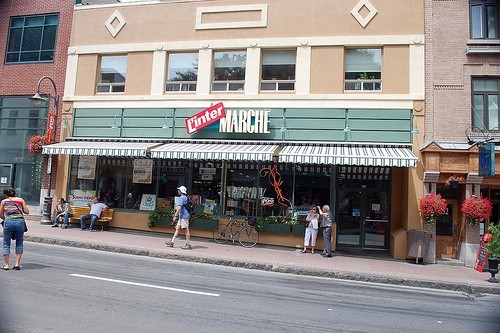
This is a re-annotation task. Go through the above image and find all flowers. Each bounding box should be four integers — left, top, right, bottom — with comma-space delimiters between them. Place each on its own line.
445, 175, 464, 186
461, 195, 493, 226
420, 193, 447, 225
29, 136, 48, 153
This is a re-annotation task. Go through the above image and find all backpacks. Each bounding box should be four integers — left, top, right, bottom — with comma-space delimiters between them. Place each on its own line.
183, 196, 195, 212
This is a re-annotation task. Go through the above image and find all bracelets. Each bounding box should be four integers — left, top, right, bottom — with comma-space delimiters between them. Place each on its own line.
174, 215, 178, 217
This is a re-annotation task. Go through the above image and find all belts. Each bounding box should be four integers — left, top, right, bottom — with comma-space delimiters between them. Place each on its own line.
13, 217, 23, 219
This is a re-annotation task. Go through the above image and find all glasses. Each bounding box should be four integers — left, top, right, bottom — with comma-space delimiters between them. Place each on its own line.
313, 209, 316, 210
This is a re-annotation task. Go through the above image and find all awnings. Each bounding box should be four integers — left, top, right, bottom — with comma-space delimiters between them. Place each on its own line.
42, 141, 163, 160
151, 143, 279, 164
279, 145, 418, 167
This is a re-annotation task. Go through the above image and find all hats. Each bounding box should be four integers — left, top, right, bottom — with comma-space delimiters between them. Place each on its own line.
177, 186, 187, 194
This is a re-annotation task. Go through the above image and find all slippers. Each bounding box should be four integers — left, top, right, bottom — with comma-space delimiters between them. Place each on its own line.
0, 265, 9, 270
13, 265, 20, 269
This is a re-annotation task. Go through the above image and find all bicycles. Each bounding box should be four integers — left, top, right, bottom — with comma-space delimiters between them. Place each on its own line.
212, 214, 259, 248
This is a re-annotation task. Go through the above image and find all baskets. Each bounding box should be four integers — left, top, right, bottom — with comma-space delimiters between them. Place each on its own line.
247, 217, 256, 225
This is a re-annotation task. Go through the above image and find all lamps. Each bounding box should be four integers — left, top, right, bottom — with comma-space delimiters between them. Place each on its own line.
162, 112, 174, 128
412, 115, 420, 134
281, 113, 287, 131
343, 111, 351, 132
62, 113, 73, 128
112, 113, 122, 129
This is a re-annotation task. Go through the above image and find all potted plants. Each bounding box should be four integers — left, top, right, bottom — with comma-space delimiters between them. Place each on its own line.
290, 218, 322, 234
147, 208, 219, 230
256, 216, 291, 233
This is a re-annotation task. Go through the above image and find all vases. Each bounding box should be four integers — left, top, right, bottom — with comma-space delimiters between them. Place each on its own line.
449, 180, 459, 189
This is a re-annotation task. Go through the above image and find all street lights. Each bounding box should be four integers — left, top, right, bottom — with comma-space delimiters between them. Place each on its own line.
28, 76, 58, 225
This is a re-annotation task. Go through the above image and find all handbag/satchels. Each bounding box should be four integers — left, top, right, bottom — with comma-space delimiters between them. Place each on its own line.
305, 221, 309, 227
24, 222, 28, 232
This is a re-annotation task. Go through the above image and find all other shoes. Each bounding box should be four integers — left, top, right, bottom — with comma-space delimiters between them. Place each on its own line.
302, 251, 306, 253
80, 228, 86, 231
52, 225, 58, 227
323, 255, 331, 257
181, 244, 191, 249
321, 253, 325, 255
164, 242, 174, 247
64, 226, 67, 229
89, 229, 93, 232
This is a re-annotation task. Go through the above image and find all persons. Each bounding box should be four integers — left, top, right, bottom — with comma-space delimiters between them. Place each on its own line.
317, 205, 332, 257
0, 188, 29, 270
51, 198, 73, 228
301, 205, 319, 255
165, 185, 191, 249
99, 188, 122, 208
79, 197, 109, 232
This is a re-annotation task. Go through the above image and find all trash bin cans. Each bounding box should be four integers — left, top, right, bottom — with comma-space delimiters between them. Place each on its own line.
407, 229, 432, 259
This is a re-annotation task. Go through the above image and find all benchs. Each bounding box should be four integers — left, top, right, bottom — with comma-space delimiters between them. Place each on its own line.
59, 207, 114, 231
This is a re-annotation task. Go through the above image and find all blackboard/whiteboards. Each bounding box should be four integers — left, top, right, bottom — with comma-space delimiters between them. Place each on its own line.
474, 241, 490, 272
